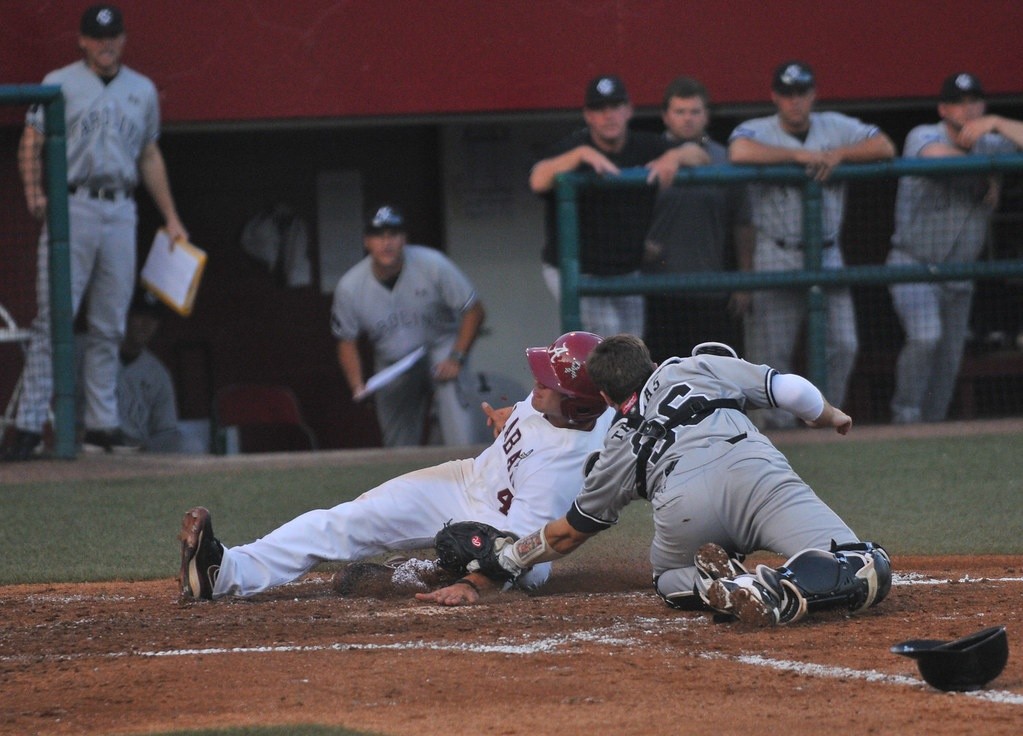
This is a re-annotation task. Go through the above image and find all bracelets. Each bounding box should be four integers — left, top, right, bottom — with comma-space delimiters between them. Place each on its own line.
448, 351, 465, 366
457, 579, 481, 597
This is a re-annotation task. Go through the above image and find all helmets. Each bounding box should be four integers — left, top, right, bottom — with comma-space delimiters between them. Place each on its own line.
525, 331, 608, 421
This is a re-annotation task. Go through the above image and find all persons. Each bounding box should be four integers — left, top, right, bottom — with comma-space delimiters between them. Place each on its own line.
466, 334, 892, 628
889, 72, 1023, 424
530, 75, 752, 367
727, 59, 897, 434
0, 5, 187, 462
178, 331, 619, 609
332, 203, 489, 449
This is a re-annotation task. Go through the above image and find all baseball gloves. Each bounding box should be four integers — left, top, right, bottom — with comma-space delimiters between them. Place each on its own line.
432, 519, 533, 585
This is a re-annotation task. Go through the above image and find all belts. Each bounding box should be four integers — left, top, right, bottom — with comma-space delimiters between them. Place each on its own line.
664, 434, 747, 477
68, 186, 134, 201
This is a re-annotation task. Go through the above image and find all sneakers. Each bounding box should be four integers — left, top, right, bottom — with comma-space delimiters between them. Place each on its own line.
694, 543, 778, 629
176, 505, 224, 603
331, 555, 408, 591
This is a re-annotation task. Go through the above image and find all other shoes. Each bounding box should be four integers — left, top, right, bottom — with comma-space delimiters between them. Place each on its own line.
89, 427, 143, 455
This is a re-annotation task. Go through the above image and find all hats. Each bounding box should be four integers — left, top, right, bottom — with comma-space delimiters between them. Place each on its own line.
940, 71, 986, 103
585, 74, 629, 106
367, 204, 407, 234
890, 627, 1008, 690
81, 4, 124, 39
771, 60, 818, 94
